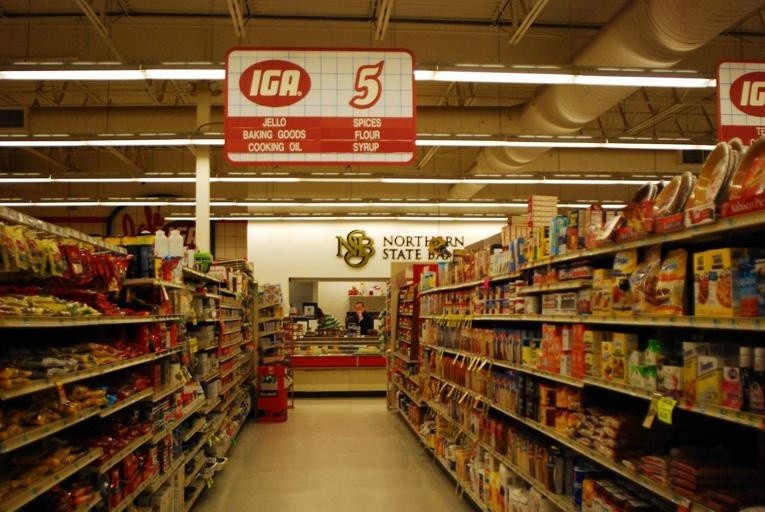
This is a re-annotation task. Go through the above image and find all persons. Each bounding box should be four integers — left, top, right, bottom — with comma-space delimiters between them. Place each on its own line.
345, 302, 370, 335
315, 309, 341, 329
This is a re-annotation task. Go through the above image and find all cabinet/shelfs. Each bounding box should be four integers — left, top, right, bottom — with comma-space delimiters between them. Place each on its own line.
1, 198, 289, 512
380, 207, 764, 512
289, 337, 388, 399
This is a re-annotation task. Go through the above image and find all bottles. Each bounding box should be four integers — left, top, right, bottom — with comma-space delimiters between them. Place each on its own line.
737, 345, 765, 415
347, 322, 357, 338
152, 229, 184, 258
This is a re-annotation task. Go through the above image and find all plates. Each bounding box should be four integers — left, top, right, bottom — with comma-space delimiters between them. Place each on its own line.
623, 132, 765, 220
